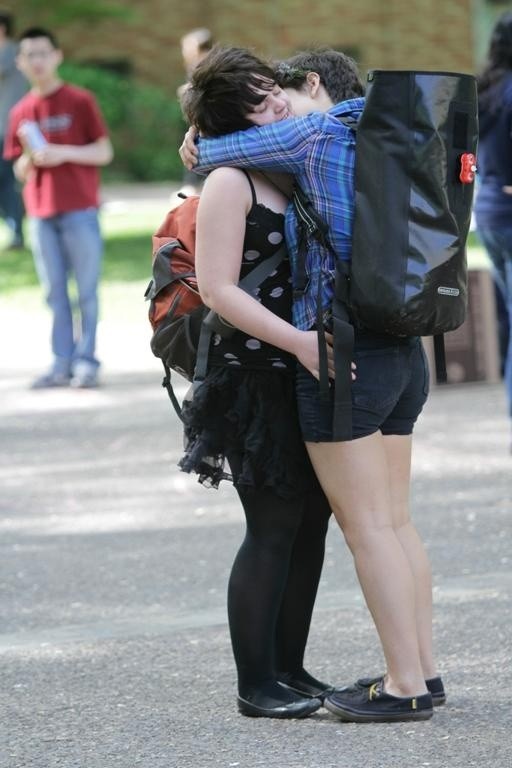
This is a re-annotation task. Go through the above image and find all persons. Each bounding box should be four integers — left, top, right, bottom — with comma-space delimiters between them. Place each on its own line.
0, 12, 31, 248
178, 45, 357, 719
3, 28, 114, 389
174, 28, 212, 198
178, 47, 447, 720
473, 11, 512, 422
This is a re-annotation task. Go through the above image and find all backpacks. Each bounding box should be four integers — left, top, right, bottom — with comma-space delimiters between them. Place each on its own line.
144, 186, 257, 388
337, 71, 480, 337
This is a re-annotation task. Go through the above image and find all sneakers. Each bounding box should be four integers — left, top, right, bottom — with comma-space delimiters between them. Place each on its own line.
238, 671, 445, 720
